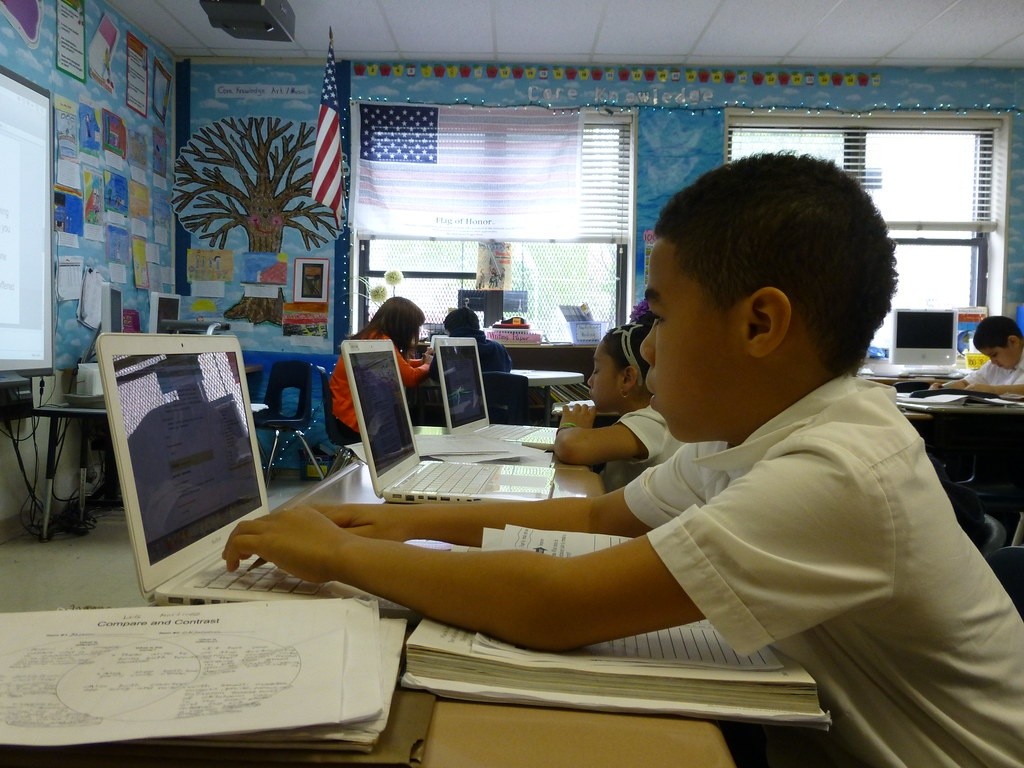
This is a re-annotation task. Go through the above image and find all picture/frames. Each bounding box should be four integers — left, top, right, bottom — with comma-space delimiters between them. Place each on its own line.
293, 257, 330, 303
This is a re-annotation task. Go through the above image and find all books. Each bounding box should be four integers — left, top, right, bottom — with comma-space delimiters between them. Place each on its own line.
400, 524, 833, 731
137, 615, 407, 753
543, 382, 591, 403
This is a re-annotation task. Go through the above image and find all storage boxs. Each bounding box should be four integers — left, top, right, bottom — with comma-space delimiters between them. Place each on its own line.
300, 460, 344, 480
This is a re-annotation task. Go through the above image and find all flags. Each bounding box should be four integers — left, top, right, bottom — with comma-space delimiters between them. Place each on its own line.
352, 99, 583, 241
311, 39, 343, 229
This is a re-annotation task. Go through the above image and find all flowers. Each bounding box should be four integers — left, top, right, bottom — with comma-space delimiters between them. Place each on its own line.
368, 284, 388, 303
384, 267, 405, 287
630, 299, 649, 323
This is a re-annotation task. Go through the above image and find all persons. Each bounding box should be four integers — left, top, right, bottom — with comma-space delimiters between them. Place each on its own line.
553, 322, 685, 495
429, 308, 512, 425
929, 316, 1024, 400
477, 266, 505, 289
328, 297, 435, 447
221, 151, 1024, 768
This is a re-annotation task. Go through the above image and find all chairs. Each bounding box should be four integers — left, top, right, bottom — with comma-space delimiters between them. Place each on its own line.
480, 372, 530, 425
892, 382, 944, 392
253, 361, 326, 487
316, 364, 353, 477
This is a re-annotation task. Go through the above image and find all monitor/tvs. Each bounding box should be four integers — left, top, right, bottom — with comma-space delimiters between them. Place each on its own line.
890, 308, 959, 376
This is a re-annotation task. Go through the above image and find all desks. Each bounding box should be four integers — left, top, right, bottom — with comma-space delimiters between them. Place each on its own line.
897, 392, 1024, 546
270, 456, 736, 768
866, 375, 958, 385
36, 403, 105, 543
420, 369, 585, 427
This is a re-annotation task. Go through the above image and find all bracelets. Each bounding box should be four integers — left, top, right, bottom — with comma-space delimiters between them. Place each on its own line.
556, 423, 577, 436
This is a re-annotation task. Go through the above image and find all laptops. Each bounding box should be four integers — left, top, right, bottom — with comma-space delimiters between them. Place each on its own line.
337, 339, 557, 507
431, 336, 562, 450
94, 331, 457, 626
